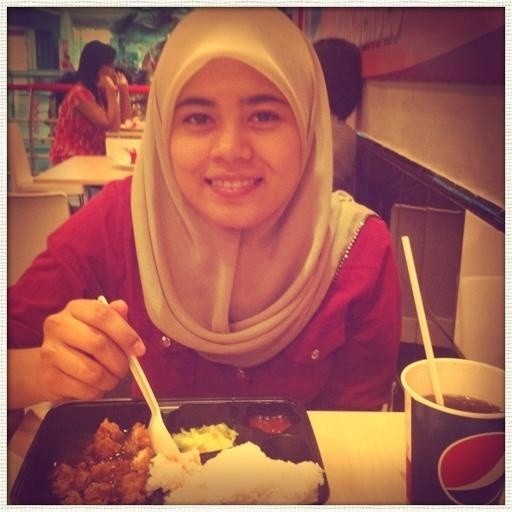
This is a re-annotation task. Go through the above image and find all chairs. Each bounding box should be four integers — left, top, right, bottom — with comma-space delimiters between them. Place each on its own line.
8, 120, 85, 291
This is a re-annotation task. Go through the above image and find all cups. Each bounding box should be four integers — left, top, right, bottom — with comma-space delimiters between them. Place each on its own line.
401, 357, 504, 503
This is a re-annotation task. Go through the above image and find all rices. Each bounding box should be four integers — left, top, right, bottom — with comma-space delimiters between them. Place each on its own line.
146, 442, 325, 505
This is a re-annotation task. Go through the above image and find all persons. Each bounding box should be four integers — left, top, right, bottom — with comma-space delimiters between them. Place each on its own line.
51, 40, 131, 167
9, 7, 403, 409
311, 37, 363, 193
